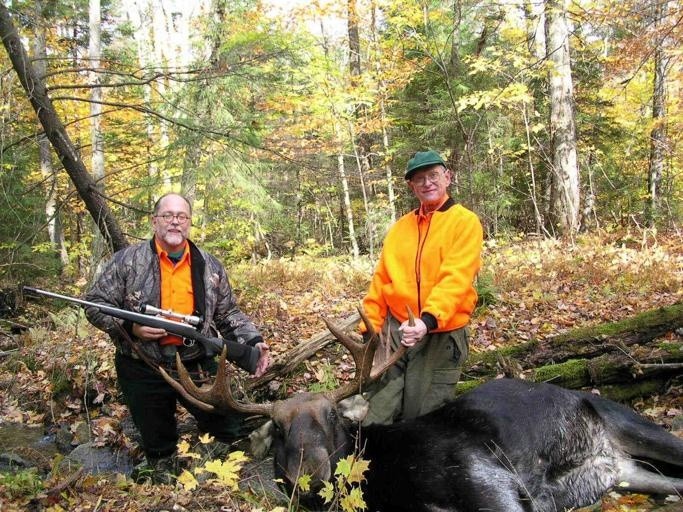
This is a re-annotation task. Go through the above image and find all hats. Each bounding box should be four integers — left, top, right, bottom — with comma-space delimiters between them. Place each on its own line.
404, 150, 447, 179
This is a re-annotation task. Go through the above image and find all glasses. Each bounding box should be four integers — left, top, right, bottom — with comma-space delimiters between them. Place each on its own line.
156, 212, 191, 224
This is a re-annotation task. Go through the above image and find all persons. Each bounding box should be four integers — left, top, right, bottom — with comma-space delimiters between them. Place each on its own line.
357, 149, 484, 426
84, 191, 271, 484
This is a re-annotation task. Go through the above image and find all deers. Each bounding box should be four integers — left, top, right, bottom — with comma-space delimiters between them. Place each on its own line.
158, 306, 683, 512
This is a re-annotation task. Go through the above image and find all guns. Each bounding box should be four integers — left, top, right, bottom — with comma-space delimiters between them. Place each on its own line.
23, 285, 259, 376
